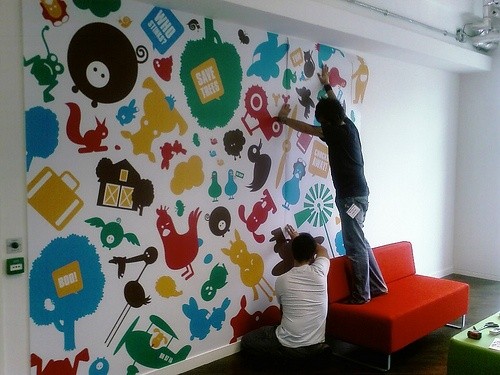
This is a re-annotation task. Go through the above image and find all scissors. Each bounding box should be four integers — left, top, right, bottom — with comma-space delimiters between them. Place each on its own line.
476, 321, 499, 331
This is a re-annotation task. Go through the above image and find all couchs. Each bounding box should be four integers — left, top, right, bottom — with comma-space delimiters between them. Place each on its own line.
325, 242, 470, 371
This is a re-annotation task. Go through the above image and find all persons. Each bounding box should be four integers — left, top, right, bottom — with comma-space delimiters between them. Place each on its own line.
240, 224, 330, 375
279, 64, 388, 304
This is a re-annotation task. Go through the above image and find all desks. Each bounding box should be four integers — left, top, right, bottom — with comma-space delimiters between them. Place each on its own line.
447, 311, 500, 375
